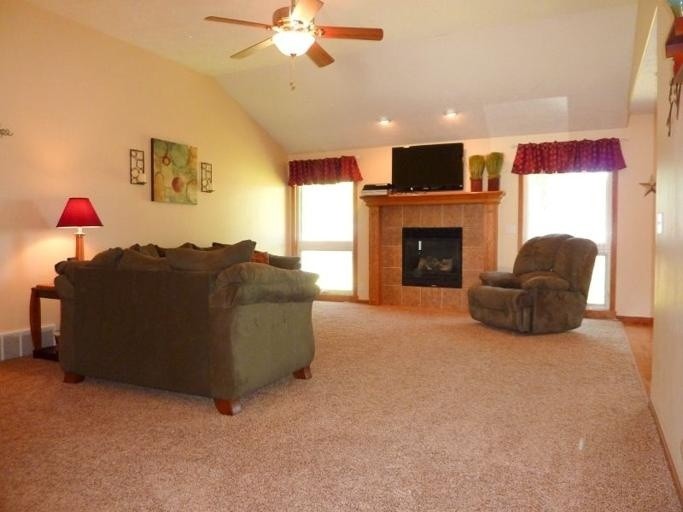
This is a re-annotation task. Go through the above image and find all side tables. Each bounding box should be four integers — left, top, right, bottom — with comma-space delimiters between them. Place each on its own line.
30, 285, 62, 362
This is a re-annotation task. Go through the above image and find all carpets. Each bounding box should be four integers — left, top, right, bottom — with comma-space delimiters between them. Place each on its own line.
0, 300, 683, 512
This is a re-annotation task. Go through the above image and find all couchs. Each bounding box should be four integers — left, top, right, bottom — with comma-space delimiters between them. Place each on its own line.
54, 248, 320, 416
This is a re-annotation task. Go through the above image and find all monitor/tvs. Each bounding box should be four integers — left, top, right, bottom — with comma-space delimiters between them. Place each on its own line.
392, 142, 464, 192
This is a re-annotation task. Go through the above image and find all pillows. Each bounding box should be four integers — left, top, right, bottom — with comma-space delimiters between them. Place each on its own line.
520, 272, 570, 290
86, 239, 301, 271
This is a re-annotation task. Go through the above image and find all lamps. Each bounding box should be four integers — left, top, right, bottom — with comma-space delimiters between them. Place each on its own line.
272, 25, 317, 90
56, 198, 104, 262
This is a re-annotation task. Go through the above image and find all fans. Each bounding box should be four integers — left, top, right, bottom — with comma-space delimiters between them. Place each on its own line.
203, 0, 384, 69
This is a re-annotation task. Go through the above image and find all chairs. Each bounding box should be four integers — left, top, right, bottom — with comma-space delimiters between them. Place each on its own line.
467, 234, 598, 335
